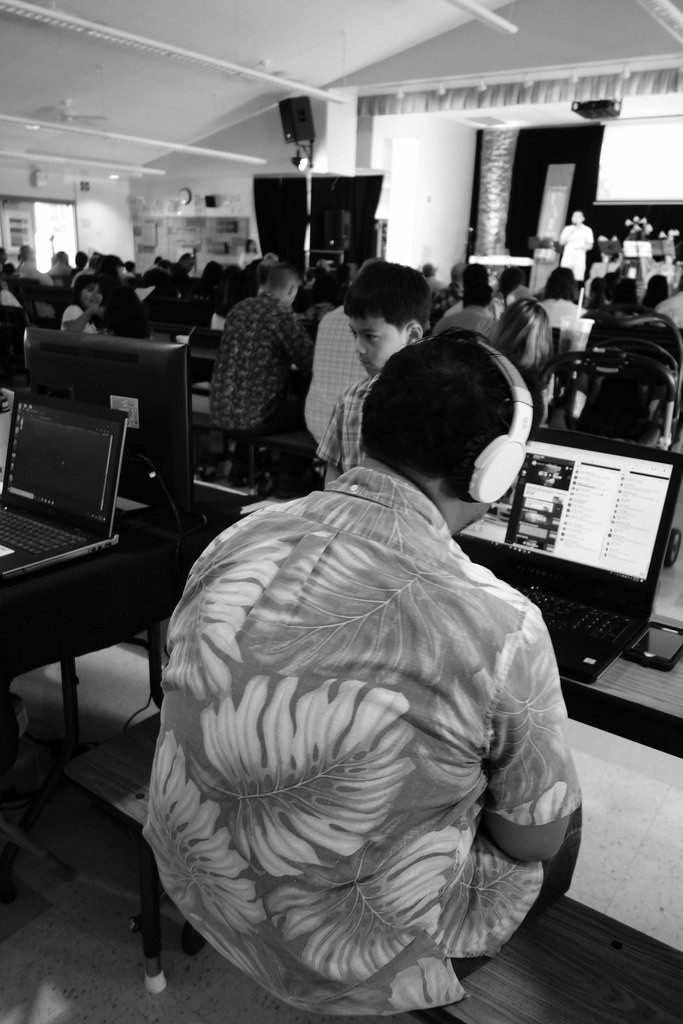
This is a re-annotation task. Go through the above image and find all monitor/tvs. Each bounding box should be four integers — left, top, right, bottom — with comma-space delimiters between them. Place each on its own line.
23, 326, 192, 513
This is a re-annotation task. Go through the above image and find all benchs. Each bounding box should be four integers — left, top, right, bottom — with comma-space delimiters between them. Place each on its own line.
190, 410, 321, 500
64, 710, 683, 1023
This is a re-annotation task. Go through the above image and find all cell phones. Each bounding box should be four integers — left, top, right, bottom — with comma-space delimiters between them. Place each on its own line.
622, 621, 682, 670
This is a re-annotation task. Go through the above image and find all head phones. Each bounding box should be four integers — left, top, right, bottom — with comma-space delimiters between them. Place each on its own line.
408, 331, 533, 504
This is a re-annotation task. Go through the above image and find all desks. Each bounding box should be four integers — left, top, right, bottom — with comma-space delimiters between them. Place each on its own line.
1, 517, 188, 901
560, 613, 683, 762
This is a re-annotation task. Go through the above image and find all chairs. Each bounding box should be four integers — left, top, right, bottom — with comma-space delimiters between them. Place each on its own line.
536, 303, 683, 452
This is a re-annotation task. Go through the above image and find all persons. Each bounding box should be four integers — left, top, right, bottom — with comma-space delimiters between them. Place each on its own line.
208, 262, 315, 497
541, 473, 555, 487
3, 242, 682, 448
143, 327, 589, 1017
316, 260, 433, 490
559, 212, 594, 287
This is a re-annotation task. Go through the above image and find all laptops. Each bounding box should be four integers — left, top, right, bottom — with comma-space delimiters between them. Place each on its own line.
1, 389, 128, 581
453, 425, 683, 684
